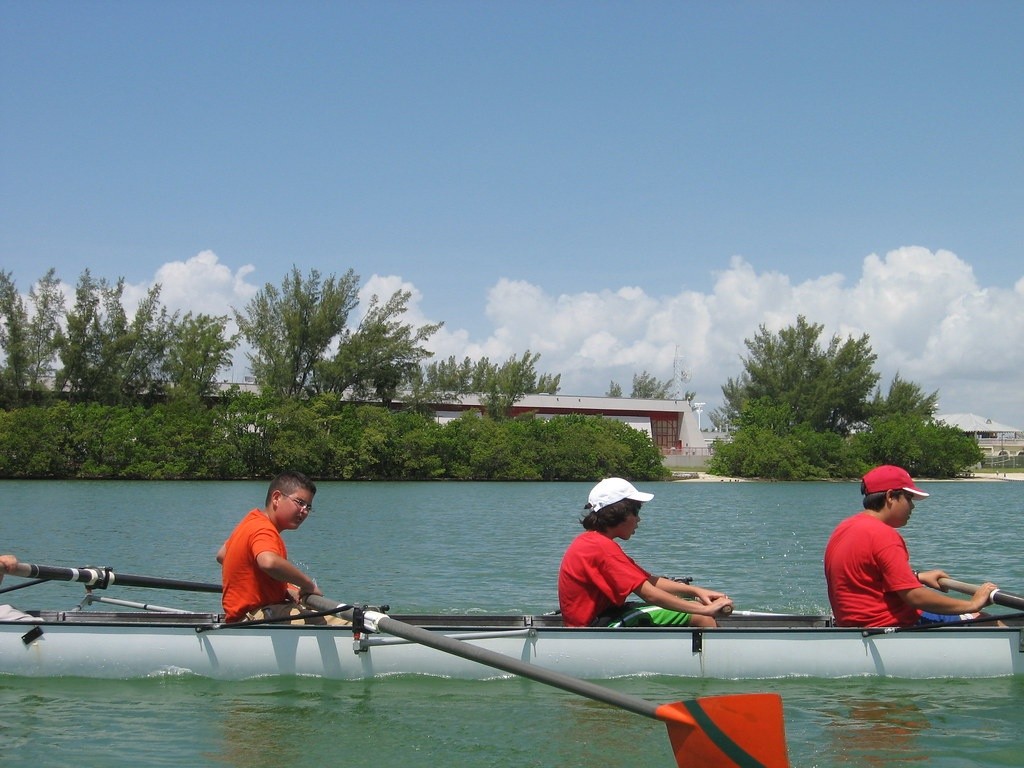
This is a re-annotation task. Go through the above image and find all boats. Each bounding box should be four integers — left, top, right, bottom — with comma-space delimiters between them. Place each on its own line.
1, 596, 1024, 680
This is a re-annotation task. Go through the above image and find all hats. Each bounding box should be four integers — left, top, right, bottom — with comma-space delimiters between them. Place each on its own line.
588, 477, 654, 513
862, 465, 929, 501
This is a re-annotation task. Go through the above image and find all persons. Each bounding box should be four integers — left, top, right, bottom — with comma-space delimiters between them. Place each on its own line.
216, 471, 351, 627
0, 554, 18, 585
825, 465, 1012, 628
558, 477, 735, 627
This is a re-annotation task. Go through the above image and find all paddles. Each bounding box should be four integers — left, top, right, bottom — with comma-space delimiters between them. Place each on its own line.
911, 568, 1024, 612
0, 559, 223, 595
285, 587, 791, 768
667, 576, 732, 616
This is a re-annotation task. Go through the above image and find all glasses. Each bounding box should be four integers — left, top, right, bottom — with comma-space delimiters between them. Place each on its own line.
281, 492, 315, 513
628, 508, 638, 516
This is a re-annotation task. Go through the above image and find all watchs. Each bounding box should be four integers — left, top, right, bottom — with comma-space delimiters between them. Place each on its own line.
912, 569, 920, 576
